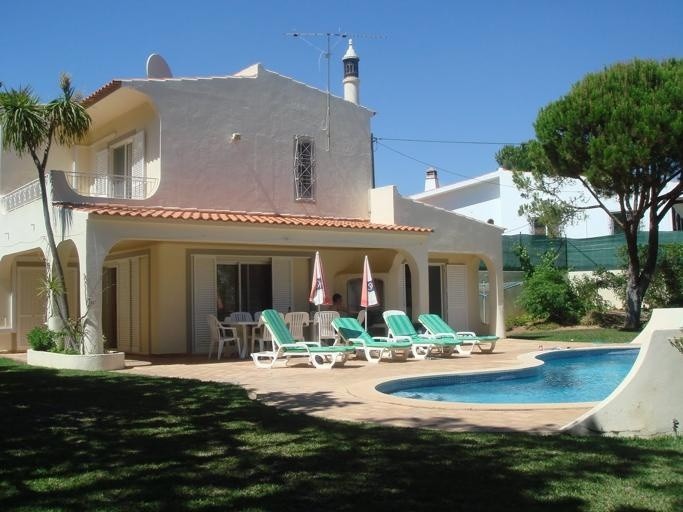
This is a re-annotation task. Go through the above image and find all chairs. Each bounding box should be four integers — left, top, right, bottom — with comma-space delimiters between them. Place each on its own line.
207, 307, 501, 372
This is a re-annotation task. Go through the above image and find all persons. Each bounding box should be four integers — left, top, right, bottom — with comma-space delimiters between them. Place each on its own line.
327, 293, 351, 313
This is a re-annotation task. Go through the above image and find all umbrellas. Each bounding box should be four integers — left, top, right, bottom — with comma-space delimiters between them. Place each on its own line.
358, 254, 380, 332
308, 251, 333, 348
